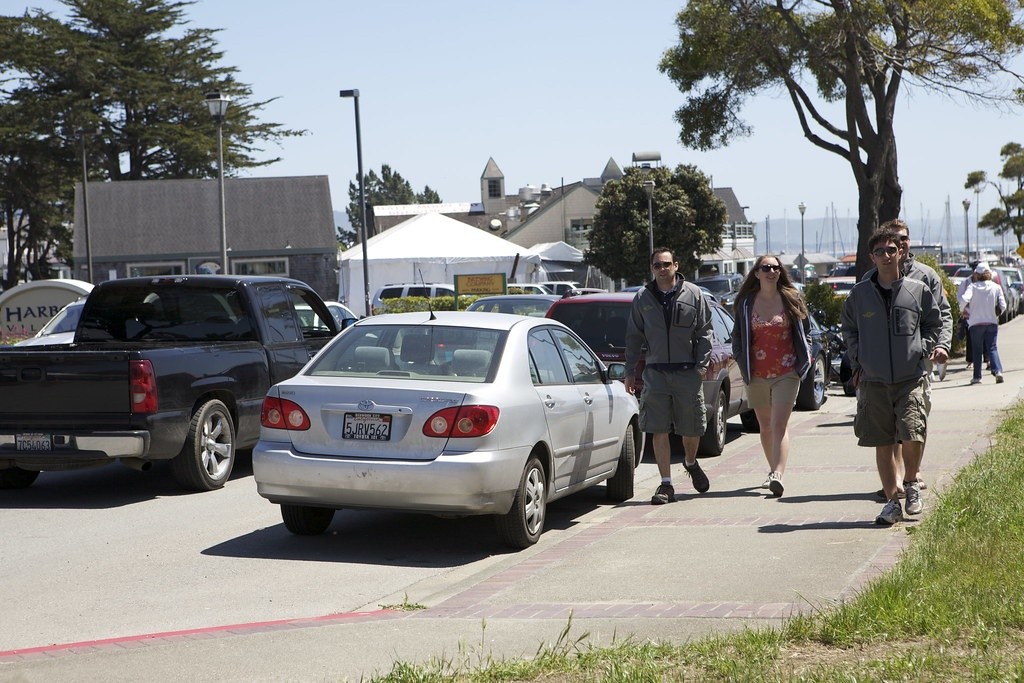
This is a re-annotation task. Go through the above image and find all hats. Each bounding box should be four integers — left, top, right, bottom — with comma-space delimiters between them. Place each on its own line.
974, 262, 991, 274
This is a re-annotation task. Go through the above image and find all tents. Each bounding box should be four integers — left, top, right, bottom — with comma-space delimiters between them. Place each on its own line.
338, 211, 541, 319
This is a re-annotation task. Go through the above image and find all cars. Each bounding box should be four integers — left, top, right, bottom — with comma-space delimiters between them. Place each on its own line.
250, 309, 649, 548
294, 301, 364, 336
11, 294, 89, 349
464, 275, 856, 458
938, 261, 1024, 326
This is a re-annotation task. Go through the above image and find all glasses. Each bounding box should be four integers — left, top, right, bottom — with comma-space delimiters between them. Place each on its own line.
871, 246, 899, 258
652, 261, 675, 270
898, 236, 911, 243
757, 264, 782, 273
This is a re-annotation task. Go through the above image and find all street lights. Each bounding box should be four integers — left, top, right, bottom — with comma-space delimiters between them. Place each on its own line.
204, 92, 233, 276
641, 179, 656, 274
338, 88, 373, 317
962, 197, 971, 264
799, 200, 808, 256
75, 126, 105, 288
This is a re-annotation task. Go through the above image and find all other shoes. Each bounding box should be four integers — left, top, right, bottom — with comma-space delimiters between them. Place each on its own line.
995, 371, 1004, 383
937, 362, 947, 381
876, 486, 906, 499
986, 361, 991, 370
971, 377, 980, 383
966, 363, 973, 369
915, 472, 927, 489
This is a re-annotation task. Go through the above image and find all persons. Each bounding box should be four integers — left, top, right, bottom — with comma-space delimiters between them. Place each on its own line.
624, 247, 715, 503
957, 261, 1006, 382
877, 219, 953, 499
840, 228, 943, 524
729, 255, 813, 496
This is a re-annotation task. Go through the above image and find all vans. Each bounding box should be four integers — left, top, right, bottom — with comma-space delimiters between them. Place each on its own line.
370, 282, 464, 317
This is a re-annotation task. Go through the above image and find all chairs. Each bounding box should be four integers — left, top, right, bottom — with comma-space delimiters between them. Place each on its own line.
452, 348, 491, 376
354, 346, 389, 372
603, 317, 629, 345
578, 319, 608, 349
398, 335, 443, 373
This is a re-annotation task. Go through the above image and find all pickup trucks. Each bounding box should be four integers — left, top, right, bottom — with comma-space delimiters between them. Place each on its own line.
0, 276, 362, 493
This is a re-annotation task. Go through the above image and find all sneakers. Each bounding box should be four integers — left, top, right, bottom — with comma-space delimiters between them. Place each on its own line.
770, 473, 784, 497
762, 472, 773, 488
903, 478, 923, 515
651, 484, 674, 504
683, 459, 710, 493
876, 499, 903, 525
